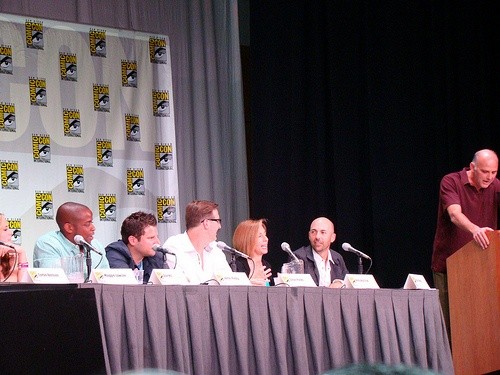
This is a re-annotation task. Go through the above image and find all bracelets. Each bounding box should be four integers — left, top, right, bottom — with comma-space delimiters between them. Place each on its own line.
18, 262, 28, 268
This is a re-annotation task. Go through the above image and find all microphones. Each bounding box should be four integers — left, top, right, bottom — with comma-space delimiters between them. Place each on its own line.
281, 242, 299, 263
152, 244, 176, 255
74, 235, 103, 255
216, 240, 252, 260
342, 243, 371, 260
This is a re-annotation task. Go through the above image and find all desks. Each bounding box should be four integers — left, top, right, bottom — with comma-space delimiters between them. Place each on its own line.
0, 281, 455, 375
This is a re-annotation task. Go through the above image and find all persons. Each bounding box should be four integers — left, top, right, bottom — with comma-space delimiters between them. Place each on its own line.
162, 200, 232, 285
229, 218, 275, 286
105, 211, 169, 285
0, 200, 30, 283
432, 149, 500, 354
33, 201, 109, 283
290, 217, 349, 290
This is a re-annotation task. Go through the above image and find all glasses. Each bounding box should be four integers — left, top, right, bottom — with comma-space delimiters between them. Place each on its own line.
201, 219, 221, 224
